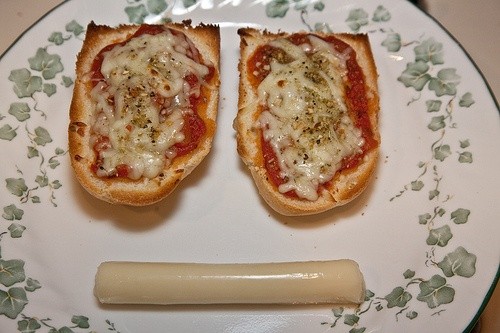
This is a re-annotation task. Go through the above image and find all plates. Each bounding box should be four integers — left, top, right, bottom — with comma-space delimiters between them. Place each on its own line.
0, 1, 500, 333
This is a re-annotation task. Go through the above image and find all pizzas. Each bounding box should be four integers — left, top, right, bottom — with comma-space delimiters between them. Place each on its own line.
232, 26, 382, 219
67, 18, 221, 208
